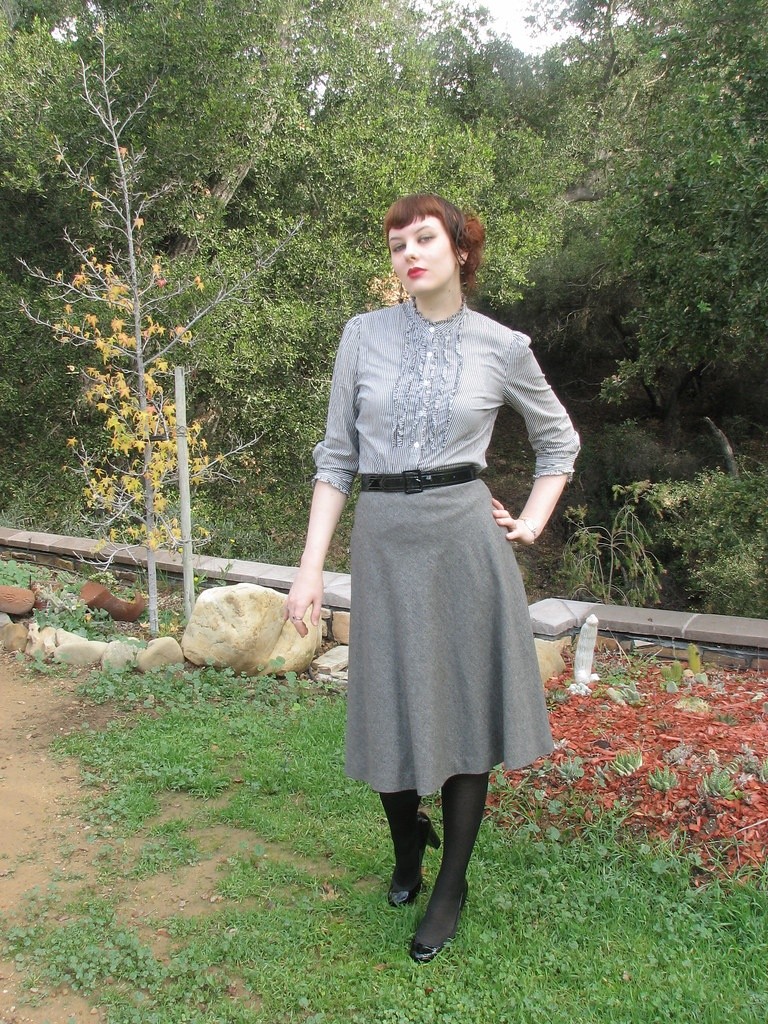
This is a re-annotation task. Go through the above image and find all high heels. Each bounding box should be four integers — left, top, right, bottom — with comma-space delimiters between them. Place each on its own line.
387, 810, 441, 907
410, 876, 469, 963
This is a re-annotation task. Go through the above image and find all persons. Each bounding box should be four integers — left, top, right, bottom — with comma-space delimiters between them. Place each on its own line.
282, 194, 581, 963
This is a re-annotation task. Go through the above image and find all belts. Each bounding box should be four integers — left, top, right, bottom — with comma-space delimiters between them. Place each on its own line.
361, 464, 477, 494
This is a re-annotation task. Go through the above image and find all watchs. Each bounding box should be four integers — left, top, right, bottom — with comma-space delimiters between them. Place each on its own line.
518, 516, 537, 546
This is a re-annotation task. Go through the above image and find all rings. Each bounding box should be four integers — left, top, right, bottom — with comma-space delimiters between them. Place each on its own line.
293, 615, 302, 620
